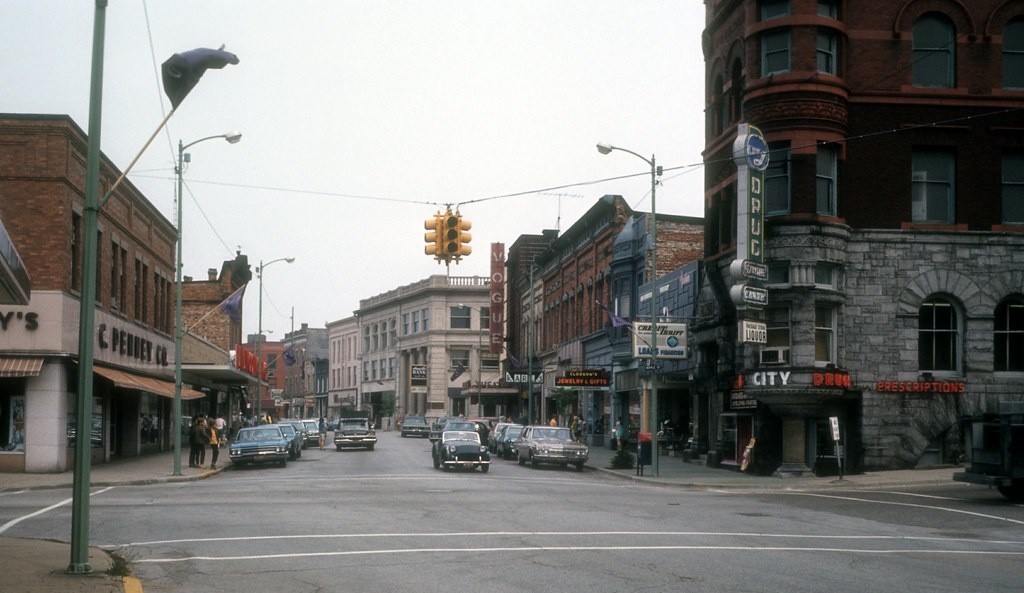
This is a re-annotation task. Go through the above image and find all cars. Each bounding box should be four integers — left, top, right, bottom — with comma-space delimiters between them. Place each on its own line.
400, 416, 431, 438
300, 420, 320, 446
486, 423, 519, 454
495, 426, 523, 461
252, 423, 304, 461
229, 425, 289, 470
512, 426, 590, 471
333, 417, 377, 451
428, 417, 498, 446
432, 431, 491, 473
283, 422, 310, 450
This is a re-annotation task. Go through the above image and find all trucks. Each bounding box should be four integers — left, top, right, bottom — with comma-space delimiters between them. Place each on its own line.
953, 413, 1024, 501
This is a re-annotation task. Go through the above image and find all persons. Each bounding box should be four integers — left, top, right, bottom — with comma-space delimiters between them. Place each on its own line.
571, 416, 583, 444
614, 416, 623, 452
549, 414, 558, 438
188, 413, 226, 470
318, 417, 326, 449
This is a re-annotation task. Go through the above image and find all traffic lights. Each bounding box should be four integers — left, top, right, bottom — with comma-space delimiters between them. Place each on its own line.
442, 216, 460, 255
425, 217, 442, 256
459, 218, 473, 256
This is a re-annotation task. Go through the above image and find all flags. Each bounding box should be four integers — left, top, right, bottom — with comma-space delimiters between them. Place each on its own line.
282, 346, 296, 366
220, 285, 244, 324
450, 364, 465, 382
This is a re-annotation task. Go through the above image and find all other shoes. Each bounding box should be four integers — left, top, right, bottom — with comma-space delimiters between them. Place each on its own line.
189, 463, 196, 467
196, 464, 206, 468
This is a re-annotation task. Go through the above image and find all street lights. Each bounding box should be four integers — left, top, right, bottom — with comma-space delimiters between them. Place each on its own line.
458, 304, 482, 418
257, 257, 295, 426
173, 131, 242, 474
597, 141, 659, 479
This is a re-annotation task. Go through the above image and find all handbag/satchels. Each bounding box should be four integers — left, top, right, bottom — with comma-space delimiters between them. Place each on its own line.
611, 427, 616, 432
